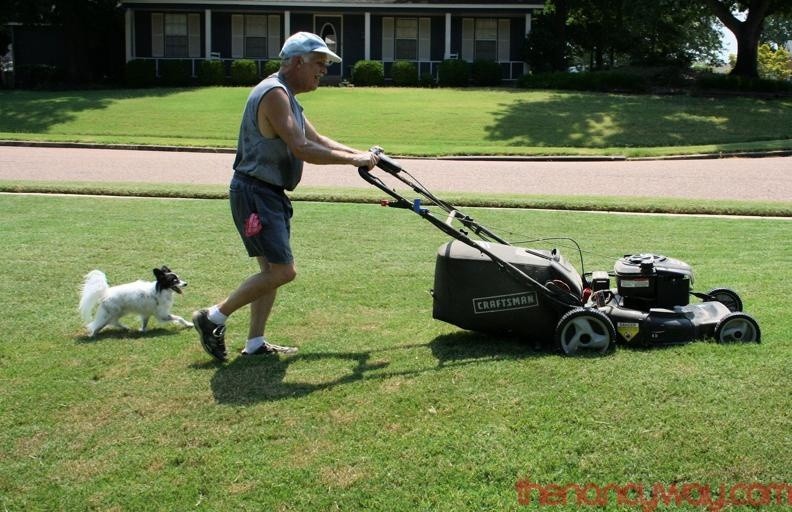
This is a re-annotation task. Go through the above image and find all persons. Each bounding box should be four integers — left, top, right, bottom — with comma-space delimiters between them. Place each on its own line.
189, 28, 383, 364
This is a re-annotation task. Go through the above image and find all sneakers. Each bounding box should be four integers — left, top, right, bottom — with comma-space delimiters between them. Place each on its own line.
192, 307, 229, 362
241, 343, 298, 355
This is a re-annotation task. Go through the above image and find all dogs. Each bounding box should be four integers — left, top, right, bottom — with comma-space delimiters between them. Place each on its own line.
77, 265, 195, 339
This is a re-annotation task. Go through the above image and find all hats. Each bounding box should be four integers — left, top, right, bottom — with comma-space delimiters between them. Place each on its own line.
279, 30, 343, 65
